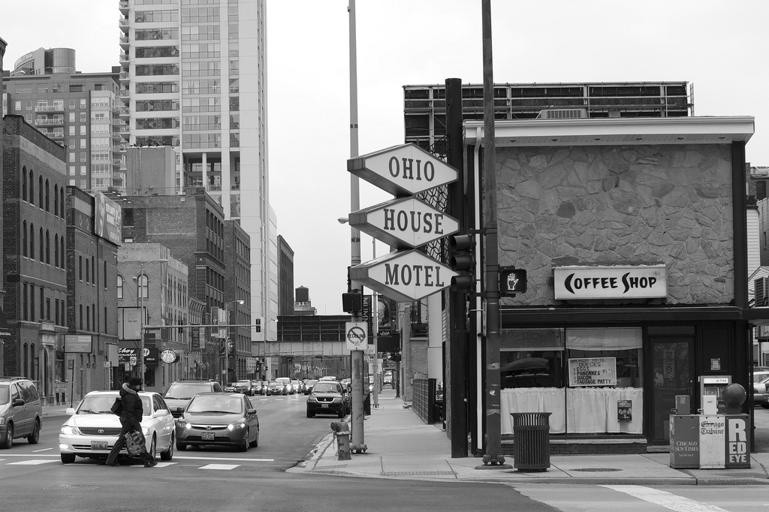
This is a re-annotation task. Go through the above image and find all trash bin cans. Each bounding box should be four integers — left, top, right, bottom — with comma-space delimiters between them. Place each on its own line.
215, 373, 220, 384
510, 412, 551, 469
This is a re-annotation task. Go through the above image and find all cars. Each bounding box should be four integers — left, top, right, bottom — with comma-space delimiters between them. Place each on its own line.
224, 375, 353, 397
175, 392, 259, 452
58, 390, 176, 464
749, 371, 769, 409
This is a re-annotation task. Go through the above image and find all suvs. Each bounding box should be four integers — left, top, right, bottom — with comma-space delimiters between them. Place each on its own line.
0, 376, 42, 449
163, 379, 223, 420
307, 381, 352, 418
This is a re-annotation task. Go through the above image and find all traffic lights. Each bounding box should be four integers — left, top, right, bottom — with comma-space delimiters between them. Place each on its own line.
342, 293, 361, 312
500, 269, 527, 293
448, 231, 477, 299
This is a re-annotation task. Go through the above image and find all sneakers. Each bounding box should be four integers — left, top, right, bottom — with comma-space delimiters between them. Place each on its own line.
144, 460, 157, 467
108, 462, 120, 466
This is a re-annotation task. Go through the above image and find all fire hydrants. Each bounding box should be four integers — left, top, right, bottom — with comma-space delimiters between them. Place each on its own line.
331, 421, 352, 461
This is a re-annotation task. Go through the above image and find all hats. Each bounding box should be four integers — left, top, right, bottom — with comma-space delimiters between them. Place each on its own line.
128, 377, 141, 385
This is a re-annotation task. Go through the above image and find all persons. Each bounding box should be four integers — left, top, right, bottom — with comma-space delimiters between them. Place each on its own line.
105, 376, 159, 468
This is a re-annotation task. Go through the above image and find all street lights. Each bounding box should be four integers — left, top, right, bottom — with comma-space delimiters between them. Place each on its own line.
225, 298, 245, 387
264, 318, 279, 382
337, 216, 378, 410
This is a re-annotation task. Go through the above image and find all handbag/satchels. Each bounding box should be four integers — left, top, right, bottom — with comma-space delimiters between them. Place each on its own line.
111, 398, 122, 416
125, 431, 145, 456
